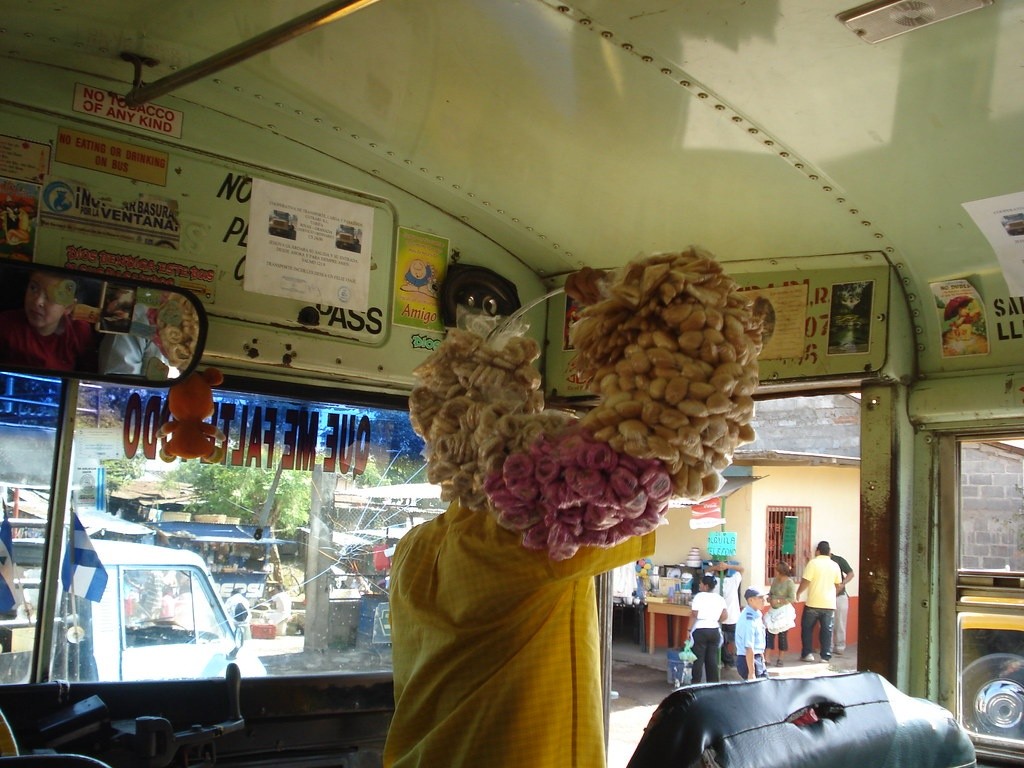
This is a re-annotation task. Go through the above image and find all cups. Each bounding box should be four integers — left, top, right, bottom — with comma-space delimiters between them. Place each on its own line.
669, 584, 689, 604
634, 599, 639, 604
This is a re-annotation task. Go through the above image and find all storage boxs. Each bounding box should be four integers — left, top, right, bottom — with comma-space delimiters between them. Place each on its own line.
658, 577, 681, 596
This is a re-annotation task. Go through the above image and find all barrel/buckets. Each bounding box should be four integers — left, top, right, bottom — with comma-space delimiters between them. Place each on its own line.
357, 594, 386, 650
666, 648, 684, 683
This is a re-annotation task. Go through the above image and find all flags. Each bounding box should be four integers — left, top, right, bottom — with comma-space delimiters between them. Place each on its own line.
0, 521, 18, 613
62, 511, 108, 603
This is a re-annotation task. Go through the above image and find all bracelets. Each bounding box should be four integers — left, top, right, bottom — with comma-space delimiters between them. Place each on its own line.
778, 600, 780, 603
687, 630, 691, 632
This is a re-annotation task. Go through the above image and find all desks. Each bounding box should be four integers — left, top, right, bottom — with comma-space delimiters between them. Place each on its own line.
648, 601, 695, 653
614, 602, 646, 654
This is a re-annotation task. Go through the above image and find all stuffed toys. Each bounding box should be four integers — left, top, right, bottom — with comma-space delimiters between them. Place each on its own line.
155, 367, 226, 463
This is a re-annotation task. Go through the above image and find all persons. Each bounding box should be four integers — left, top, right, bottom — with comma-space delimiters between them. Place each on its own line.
265, 583, 291, 625
2, 270, 180, 378
124, 570, 179, 625
225, 588, 252, 640
384, 500, 656, 768
687, 562, 745, 685
735, 588, 770, 681
365, 582, 388, 602
763, 561, 796, 667
796, 541, 854, 663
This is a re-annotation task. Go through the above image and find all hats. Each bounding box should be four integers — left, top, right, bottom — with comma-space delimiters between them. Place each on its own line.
744, 589, 766, 600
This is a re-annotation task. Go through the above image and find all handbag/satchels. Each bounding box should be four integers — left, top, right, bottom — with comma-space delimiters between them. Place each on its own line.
679, 639, 698, 661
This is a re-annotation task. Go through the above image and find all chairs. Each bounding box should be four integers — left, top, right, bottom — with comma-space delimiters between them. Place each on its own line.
0, 754, 113, 768
627, 671, 978, 768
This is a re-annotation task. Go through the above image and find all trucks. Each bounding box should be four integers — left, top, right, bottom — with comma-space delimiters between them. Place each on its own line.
0, 538, 272, 686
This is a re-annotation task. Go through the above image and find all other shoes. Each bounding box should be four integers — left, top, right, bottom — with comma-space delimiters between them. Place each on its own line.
765, 662, 771, 666
776, 659, 783, 667
800, 653, 814, 662
819, 659, 828, 663
832, 650, 843, 655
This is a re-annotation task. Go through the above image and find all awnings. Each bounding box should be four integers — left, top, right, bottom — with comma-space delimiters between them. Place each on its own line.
147, 522, 297, 543
